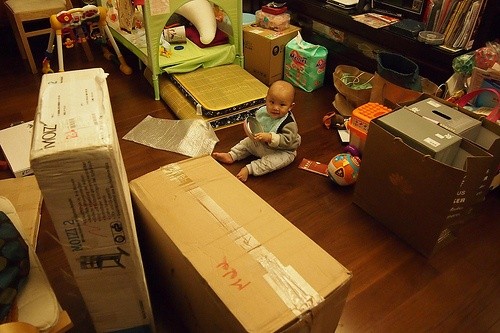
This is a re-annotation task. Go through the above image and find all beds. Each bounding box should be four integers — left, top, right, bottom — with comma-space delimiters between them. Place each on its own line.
98, 0, 243, 101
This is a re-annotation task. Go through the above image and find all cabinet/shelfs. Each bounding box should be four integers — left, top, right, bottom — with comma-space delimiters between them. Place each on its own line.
251, 0, 468, 99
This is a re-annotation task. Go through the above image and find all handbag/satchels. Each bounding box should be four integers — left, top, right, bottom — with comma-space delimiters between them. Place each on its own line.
284, 31, 328, 93
370, 71, 443, 112
353, 84, 500, 260
333, 65, 374, 107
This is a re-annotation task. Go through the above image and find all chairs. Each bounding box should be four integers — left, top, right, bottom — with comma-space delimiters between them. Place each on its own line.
3, 2, 94, 74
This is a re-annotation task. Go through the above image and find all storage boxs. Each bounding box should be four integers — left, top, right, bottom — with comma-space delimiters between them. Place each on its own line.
243, 23, 302, 88
0, 120, 36, 179
28, 67, 155, 333
127, 155, 354, 333
378, 98, 483, 168
347, 102, 392, 156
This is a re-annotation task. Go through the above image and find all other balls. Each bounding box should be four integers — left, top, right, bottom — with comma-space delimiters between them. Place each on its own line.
328, 153, 360, 187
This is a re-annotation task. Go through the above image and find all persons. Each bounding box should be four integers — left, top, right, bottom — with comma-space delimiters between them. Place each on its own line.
212, 80, 301, 182
